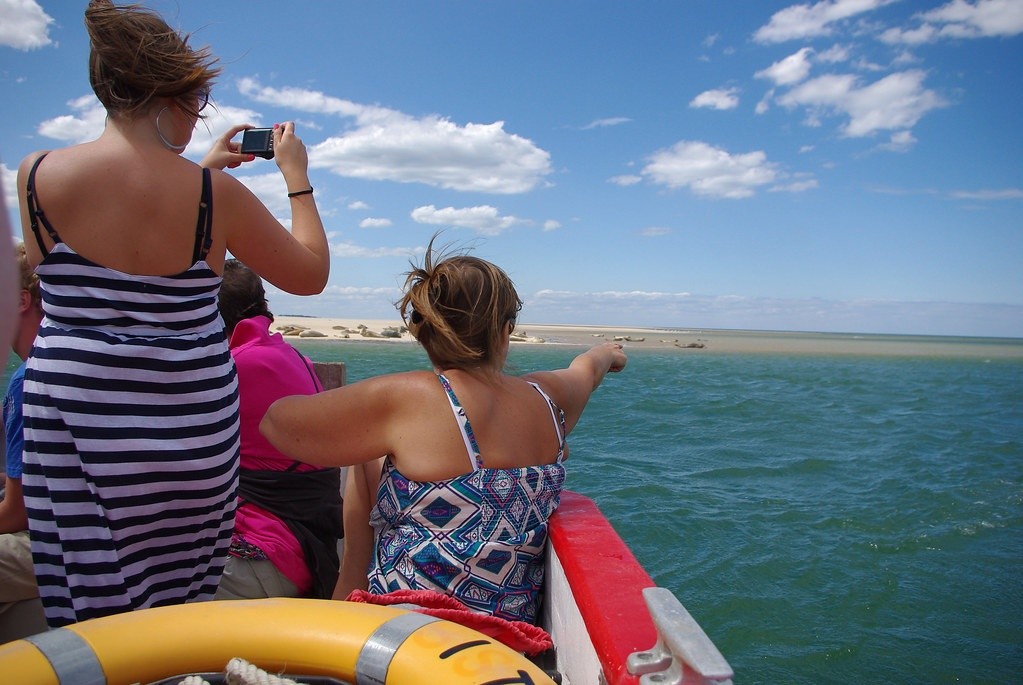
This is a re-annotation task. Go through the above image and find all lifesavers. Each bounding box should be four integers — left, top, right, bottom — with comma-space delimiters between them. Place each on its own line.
1, 594, 564, 685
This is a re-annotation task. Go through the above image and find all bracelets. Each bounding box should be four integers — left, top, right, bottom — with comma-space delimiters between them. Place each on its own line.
288, 186, 314, 200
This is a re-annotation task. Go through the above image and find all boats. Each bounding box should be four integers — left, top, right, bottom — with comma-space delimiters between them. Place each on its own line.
591, 334, 704, 348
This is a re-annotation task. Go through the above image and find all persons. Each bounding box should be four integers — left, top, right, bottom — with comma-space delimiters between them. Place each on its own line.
0, 0, 331, 645
258, 228, 628, 630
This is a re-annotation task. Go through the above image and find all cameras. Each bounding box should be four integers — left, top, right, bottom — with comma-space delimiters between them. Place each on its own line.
241, 127, 275, 160
